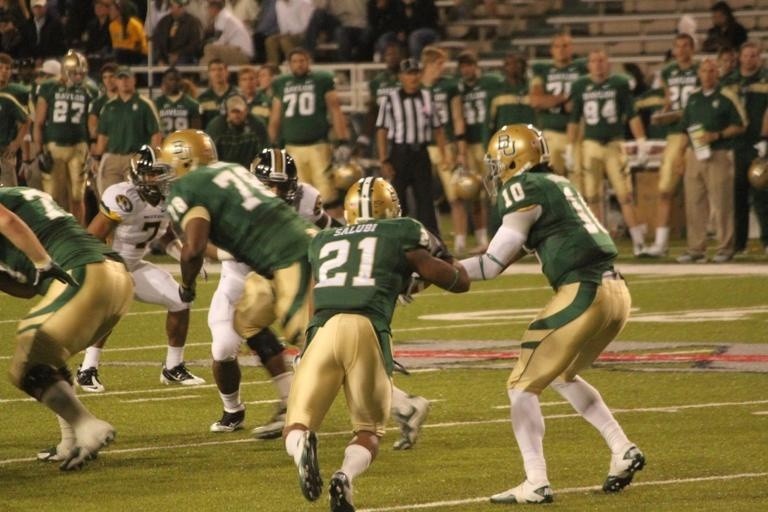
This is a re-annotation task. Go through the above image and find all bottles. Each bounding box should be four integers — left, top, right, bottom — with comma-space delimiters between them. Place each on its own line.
36, 61, 61, 77
30, 0, 47, 9
113, 64, 133, 78
226, 94, 247, 113
399, 57, 421, 75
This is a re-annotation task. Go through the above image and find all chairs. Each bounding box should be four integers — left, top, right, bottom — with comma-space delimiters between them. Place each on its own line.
390, 392, 431, 450
631, 242, 733, 263
158, 360, 207, 386
327, 471, 357, 511
73, 364, 106, 393
209, 402, 246, 433
599, 441, 645, 493
293, 429, 324, 501
250, 408, 287, 440
36, 420, 117, 472
488, 478, 553, 504
450, 243, 487, 260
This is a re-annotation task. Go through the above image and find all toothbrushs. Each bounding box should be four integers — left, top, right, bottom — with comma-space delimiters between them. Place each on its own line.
400, 228, 442, 294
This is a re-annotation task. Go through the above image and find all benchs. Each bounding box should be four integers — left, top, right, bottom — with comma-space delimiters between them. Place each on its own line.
149, 0, 768, 79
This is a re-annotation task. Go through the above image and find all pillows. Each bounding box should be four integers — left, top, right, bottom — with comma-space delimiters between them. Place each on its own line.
249, 147, 297, 205
125, 127, 219, 207
332, 159, 366, 190
61, 49, 88, 90
343, 175, 403, 225
747, 156, 767, 190
482, 124, 553, 197
455, 171, 483, 202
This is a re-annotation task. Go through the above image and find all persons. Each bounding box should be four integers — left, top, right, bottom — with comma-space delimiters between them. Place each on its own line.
1, 1, 501, 265
701, 2, 747, 54
161, 128, 428, 451
75, 144, 205, 392
282, 176, 470, 512
480, 34, 653, 258
207, 149, 346, 433
0, 202, 81, 291
459, 124, 644, 505
644, 33, 766, 264
0, 185, 135, 473
